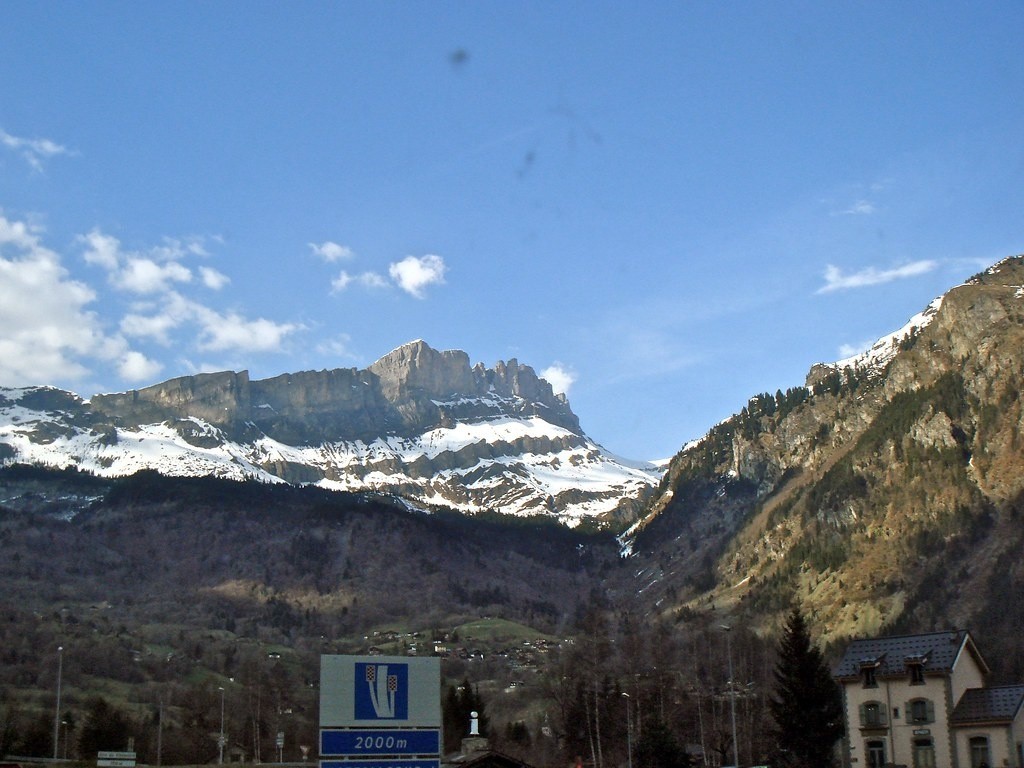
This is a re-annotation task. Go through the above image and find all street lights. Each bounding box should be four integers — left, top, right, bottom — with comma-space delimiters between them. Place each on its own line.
157, 696, 164, 767
218, 687, 225, 766
53, 645, 68, 759
622, 693, 632, 768
